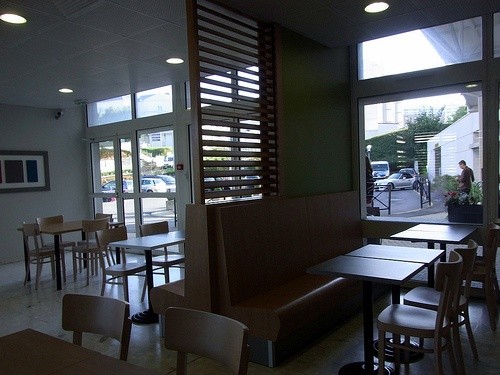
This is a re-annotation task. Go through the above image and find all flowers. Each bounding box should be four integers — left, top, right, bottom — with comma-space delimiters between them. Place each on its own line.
443, 193, 479, 207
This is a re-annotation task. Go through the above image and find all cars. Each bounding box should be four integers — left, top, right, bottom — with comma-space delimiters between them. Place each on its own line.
143, 175, 176, 192
371, 161, 390, 179
101, 180, 133, 202
400, 168, 417, 176
373, 172, 421, 192
141, 178, 167, 193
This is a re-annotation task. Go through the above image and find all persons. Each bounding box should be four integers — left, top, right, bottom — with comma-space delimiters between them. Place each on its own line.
456, 160, 474, 194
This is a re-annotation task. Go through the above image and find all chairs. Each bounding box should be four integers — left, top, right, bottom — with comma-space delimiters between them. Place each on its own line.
27, 211, 500, 375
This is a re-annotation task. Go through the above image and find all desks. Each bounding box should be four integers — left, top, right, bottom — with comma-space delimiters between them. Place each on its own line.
109, 231, 186, 324
0, 328, 167, 375
17, 220, 125, 291
345, 243, 446, 364
389, 225, 478, 289
306, 255, 425, 375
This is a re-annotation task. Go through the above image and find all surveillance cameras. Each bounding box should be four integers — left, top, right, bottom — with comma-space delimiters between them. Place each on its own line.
58, 112, 64, 116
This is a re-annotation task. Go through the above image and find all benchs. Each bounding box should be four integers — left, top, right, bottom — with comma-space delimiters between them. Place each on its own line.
150, 279, 185, 347
215, 191, 392, 369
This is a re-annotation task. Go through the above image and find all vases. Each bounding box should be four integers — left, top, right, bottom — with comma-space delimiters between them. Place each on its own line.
448, 204, 483, 223
